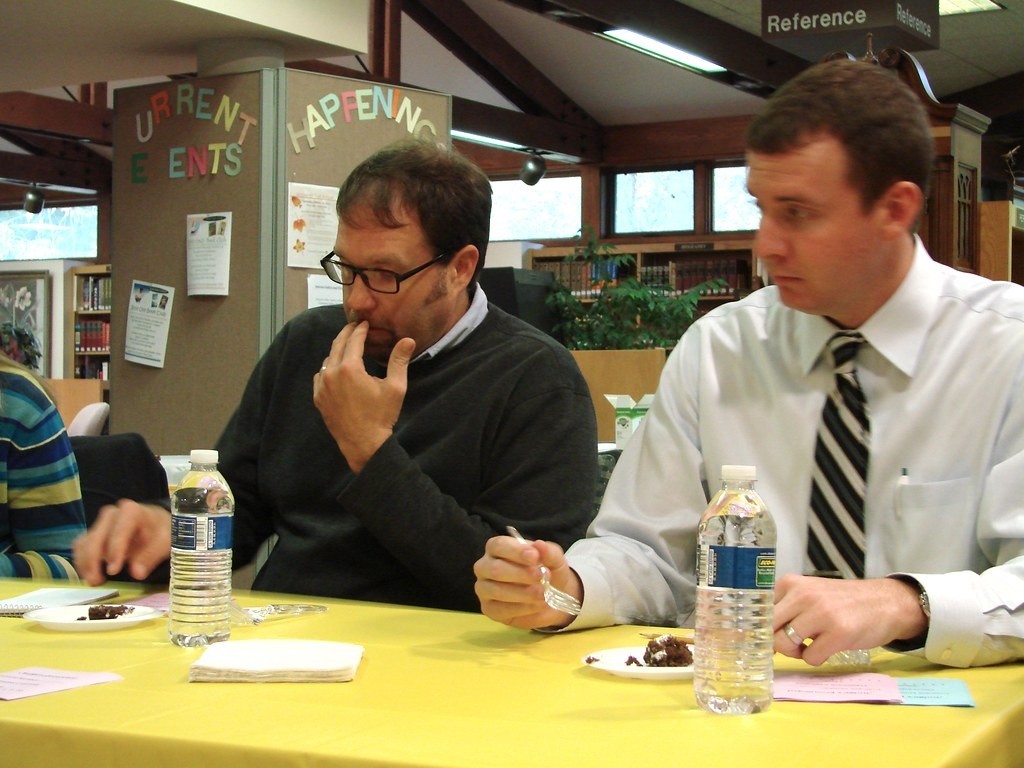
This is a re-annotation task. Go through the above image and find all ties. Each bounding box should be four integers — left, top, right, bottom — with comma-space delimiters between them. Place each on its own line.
798, 331, 871, 580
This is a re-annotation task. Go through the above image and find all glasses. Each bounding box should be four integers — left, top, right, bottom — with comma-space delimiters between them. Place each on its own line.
319, 242, 466, 294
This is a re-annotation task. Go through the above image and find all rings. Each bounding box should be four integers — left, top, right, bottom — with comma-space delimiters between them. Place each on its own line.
320, 367, 326, 371
783, 624, 803, 645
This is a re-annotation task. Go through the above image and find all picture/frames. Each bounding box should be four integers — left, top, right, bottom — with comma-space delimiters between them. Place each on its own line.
0, 270, 50, 379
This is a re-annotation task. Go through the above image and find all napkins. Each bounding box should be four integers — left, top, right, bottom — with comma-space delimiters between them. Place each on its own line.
188, 640, 363, 682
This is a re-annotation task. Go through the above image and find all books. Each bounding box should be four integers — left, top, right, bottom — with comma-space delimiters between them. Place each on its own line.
83, 276, 111, 310
0, 587, 119, 617
75, 320, 110, 352
641, 259, 747, 296
75, 361, 109, 381
535, 260, 617, 299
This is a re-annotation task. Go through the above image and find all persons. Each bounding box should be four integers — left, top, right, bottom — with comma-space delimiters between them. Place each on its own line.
473, 59, 1024, 668
73, 137, 599, 614
0, 349, 87, 581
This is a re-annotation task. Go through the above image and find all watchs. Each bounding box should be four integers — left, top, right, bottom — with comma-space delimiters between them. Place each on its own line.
919, 583, 930, 618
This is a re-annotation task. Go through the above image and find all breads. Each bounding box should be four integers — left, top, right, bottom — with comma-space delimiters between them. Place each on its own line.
88, 604, 128, 620
642, 634, 693, 667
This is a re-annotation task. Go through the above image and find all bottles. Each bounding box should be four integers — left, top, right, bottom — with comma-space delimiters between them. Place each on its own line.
167, 450, 235, 647
695, 465, 778, 713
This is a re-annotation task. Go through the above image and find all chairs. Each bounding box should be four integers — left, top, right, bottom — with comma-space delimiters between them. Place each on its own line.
69, 432, 169, 583
68, 402, 110, 437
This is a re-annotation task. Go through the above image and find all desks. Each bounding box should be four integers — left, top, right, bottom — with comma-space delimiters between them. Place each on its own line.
1, 576, 1024, 768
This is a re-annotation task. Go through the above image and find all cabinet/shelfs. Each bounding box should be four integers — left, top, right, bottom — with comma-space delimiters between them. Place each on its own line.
527, 239, 760, 349
72, 263, 111, 390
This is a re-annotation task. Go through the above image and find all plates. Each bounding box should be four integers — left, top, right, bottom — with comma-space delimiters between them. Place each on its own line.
23, 604, 162, 631
581, 644, 695, 680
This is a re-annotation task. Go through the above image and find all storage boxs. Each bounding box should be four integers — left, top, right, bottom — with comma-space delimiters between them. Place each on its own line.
604, 394, 654, 449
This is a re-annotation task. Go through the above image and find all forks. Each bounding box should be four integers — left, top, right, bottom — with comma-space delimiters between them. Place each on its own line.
507, 525, 580, 616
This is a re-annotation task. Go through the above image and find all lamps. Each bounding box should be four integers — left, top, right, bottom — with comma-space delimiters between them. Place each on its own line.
24, 182, 44, 213
520, 148, 547, 185
